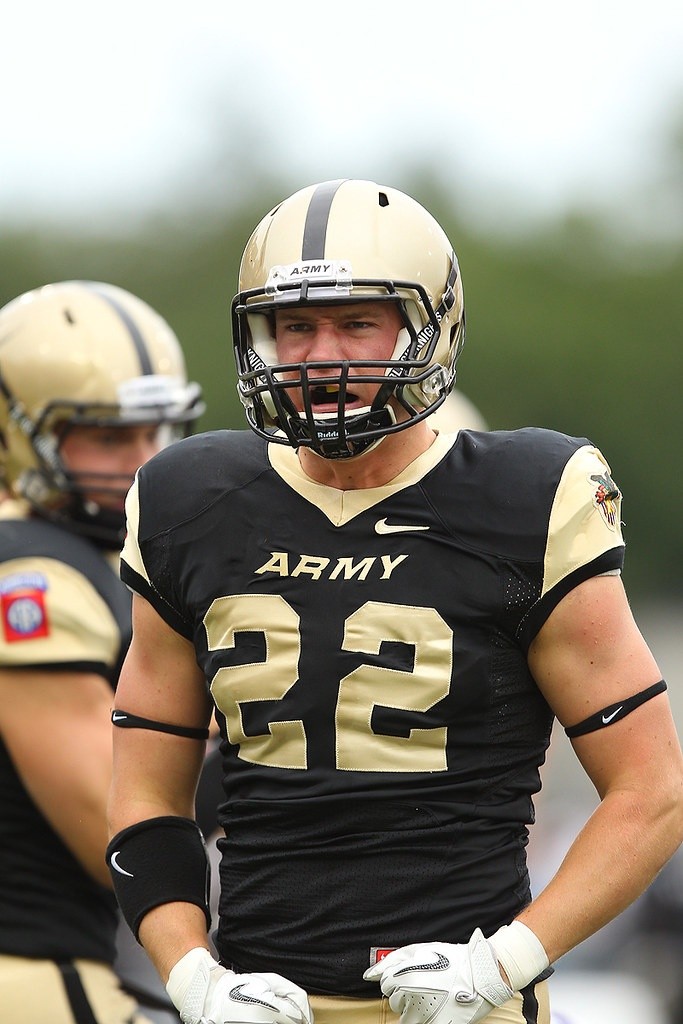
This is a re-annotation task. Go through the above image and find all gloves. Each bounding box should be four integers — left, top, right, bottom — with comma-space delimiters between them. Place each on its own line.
361, 928, 517, 1024
165, 944, 316, 1024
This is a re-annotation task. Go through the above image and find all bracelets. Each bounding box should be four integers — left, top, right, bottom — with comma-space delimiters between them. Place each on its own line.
106, 816, 212, 946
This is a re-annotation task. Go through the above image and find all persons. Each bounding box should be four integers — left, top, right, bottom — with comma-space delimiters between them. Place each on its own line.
0, 276, 210, 1024
103, 175, 683, 1023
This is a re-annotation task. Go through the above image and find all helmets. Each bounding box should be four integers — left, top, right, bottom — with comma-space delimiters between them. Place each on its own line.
0, 278, 211, 553
232, 179, 467, 459
